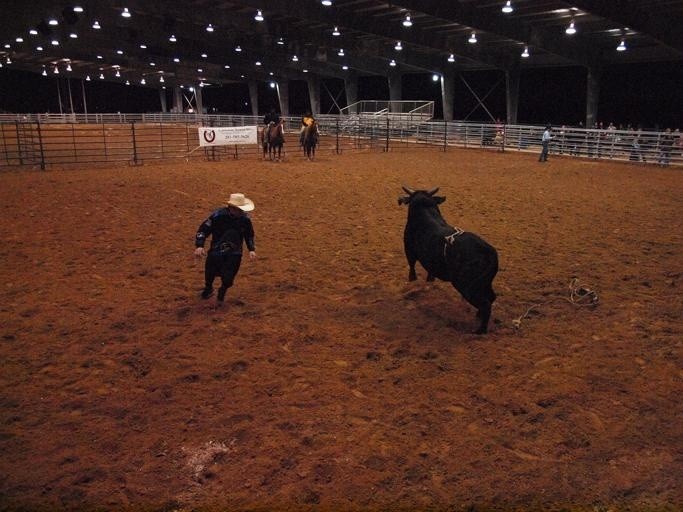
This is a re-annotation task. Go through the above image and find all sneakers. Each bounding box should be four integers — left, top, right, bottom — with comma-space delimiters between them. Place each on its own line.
203, 288, 224, 302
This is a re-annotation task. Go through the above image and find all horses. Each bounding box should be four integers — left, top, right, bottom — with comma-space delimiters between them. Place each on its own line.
301, 120, 318, 159
260, 120, 287, 160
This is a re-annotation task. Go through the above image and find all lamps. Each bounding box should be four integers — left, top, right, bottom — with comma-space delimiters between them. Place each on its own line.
0, 0, 629, 92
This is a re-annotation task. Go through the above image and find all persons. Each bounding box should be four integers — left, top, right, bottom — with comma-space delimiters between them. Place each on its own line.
297, 109, 314, 143
193, 194, 258, 302
263, 108, 284, 144
559, 122, 682, 165
537, 125, 556, 163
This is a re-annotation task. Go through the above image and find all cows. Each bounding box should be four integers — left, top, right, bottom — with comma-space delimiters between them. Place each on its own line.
395, 185, 500, 335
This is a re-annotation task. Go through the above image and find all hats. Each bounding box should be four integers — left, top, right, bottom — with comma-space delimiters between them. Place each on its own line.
226, 193, 255, 212
